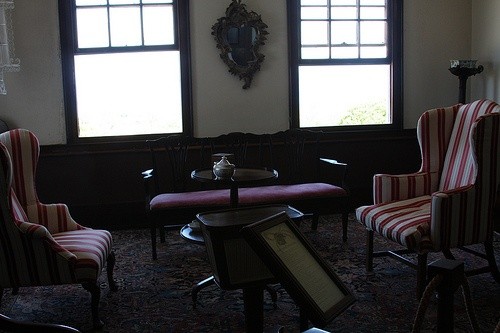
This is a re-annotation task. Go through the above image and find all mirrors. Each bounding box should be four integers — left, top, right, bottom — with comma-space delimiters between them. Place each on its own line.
211, 0, 270, 89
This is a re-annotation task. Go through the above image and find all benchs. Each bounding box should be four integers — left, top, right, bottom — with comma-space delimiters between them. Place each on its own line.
138, 127, 351, 261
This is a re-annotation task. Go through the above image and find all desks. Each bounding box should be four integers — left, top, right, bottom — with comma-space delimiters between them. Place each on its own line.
179, 165, 279, 311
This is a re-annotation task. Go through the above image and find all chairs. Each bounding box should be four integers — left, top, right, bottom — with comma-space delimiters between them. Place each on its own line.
354, 97, 500, 301
0, 128, 121, 333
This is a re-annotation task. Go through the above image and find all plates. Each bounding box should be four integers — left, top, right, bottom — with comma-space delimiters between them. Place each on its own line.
188, 221, 202, 229
